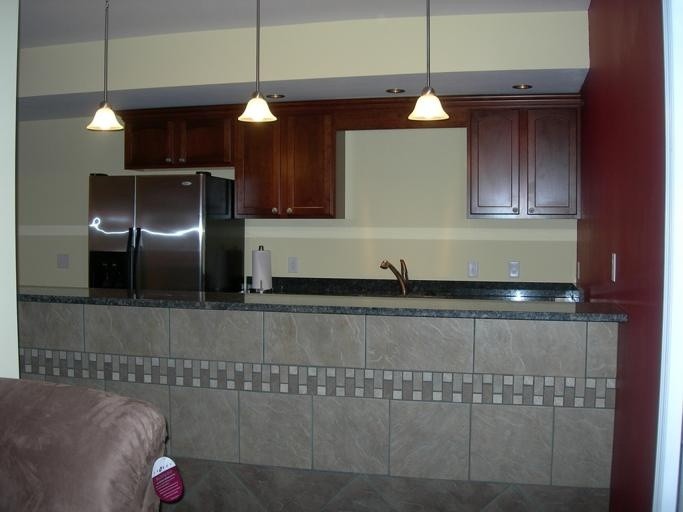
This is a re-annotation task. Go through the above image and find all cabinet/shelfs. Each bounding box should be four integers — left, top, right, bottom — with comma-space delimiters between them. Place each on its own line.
466, 93, 582, 219
233, 99, 336, 219
115, 104, 234, 169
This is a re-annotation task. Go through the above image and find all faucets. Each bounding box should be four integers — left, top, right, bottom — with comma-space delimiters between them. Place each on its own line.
380, 259, 409, 295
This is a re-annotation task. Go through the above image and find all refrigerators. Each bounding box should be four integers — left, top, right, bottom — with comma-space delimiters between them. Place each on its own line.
87, 170, 246, 303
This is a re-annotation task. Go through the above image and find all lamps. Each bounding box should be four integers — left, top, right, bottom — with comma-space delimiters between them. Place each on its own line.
87, 0, 124, 131
237, 0, 278, 123
408, 0, 449, 121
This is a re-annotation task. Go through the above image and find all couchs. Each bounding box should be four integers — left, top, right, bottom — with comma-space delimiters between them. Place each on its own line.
1, 378, 166, 511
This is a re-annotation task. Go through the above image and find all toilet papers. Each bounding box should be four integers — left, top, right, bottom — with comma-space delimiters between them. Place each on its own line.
252, 249, 272, 290
